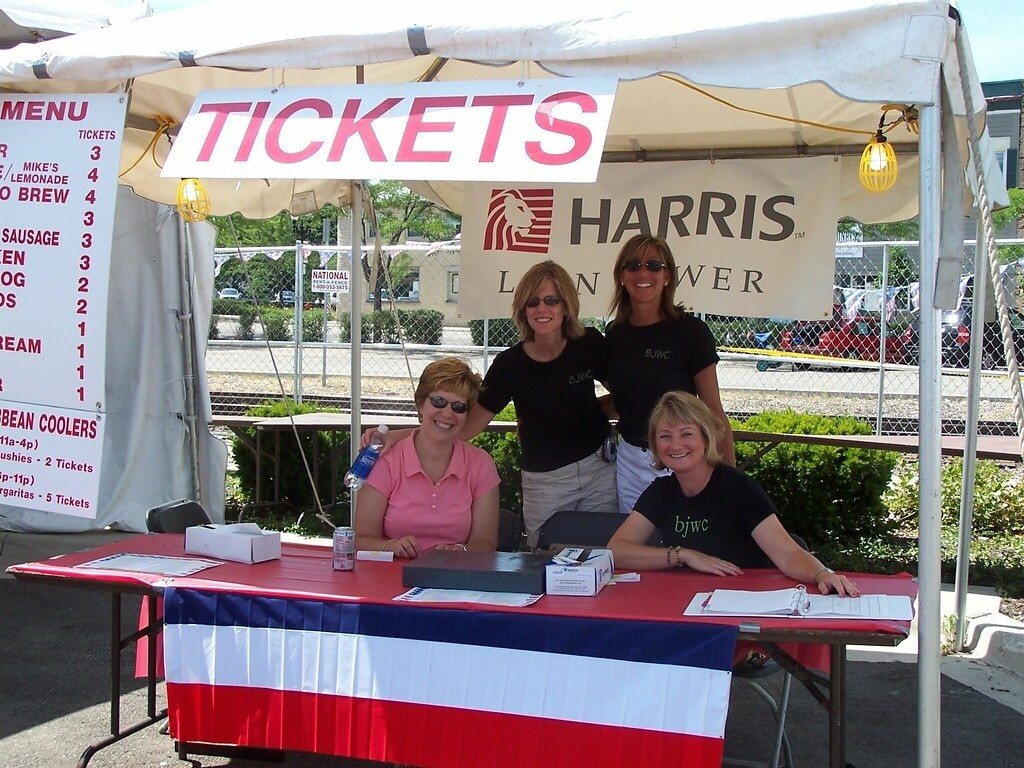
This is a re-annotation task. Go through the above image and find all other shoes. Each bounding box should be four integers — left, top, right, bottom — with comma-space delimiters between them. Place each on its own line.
742, 649, 768, 668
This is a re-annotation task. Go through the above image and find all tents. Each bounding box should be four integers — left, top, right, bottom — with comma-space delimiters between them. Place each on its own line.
0, 0, 987, 768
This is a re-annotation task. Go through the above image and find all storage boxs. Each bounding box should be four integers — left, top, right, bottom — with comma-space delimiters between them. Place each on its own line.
403, 549, 551, 595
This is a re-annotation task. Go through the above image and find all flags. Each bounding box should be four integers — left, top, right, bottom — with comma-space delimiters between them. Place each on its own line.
163, 587, 739, 768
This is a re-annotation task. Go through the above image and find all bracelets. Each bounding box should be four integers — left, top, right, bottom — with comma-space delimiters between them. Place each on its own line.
666, 546, 682, 568
456, 544, 467, 551
383, 540, 392, 551
814, 568, 835, 578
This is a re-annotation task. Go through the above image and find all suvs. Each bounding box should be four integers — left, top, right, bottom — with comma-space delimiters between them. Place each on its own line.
901, 298, 1024, 370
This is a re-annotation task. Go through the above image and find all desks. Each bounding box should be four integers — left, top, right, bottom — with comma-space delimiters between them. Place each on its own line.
209, 412, 1024, 542
7, 532, 920, 768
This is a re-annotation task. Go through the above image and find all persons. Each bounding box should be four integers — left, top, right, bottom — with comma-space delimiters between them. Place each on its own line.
605, 390, 861, 598
357, 261, 619, 550
355, 357, 502, 558
597, 234, 736, 514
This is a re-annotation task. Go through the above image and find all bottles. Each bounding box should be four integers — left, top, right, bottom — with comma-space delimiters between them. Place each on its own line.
343, 424, 389, 492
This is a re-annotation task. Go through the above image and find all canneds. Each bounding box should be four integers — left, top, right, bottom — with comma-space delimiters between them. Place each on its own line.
333, 526, 356, 570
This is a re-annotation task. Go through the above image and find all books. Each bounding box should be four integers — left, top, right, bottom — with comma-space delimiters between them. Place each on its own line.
704, 589, 801, 614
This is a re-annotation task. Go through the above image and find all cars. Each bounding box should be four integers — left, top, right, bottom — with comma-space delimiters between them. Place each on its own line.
275, 290, 294, 301
782, 317, 905, 370
217, 288, 242, 300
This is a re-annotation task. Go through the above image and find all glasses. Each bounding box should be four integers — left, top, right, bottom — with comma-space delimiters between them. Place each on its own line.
621, 259, 667, 272
426, 394, 469, 414
525, 295, 563, 306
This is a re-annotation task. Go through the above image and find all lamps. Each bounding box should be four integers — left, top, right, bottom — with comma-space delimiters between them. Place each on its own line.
157, 116, 214, 223
860, 101, 916, 190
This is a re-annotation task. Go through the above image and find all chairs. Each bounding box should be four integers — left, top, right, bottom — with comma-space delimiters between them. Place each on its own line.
722, 534, 809, 768
145, 497, 212, 533
536, 511, 659, 546
